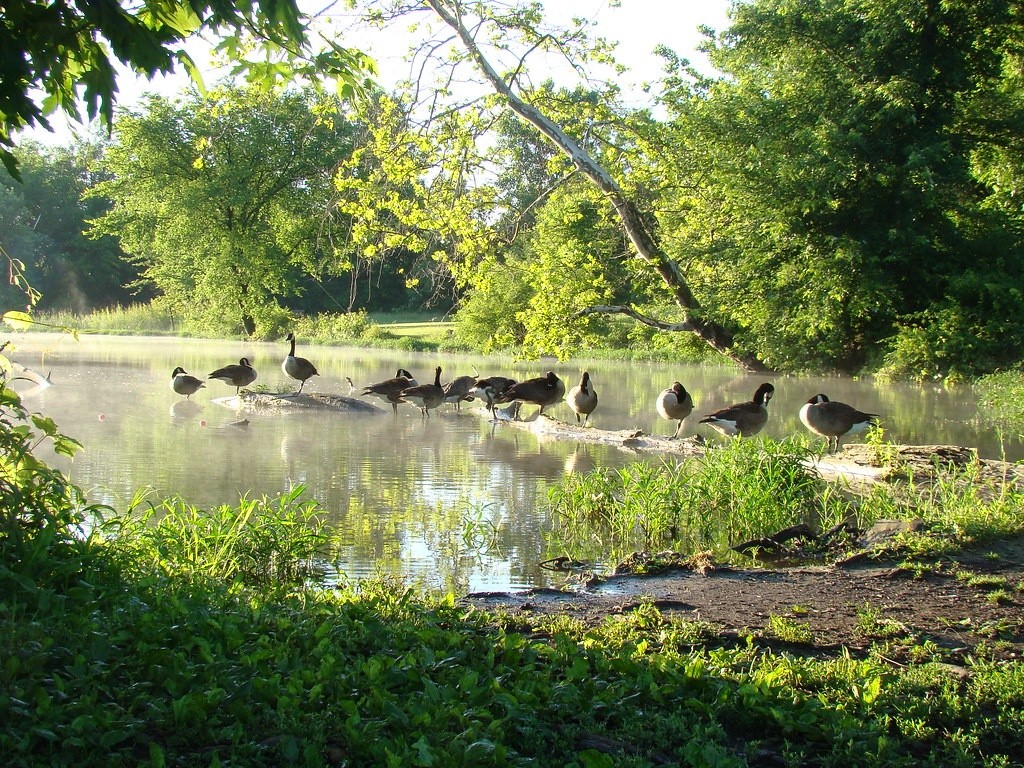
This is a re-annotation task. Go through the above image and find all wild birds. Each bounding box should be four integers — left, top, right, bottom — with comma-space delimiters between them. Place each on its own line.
281, 333, 320, 396
799, 393, 882, 451
499, 371, 566, 416
656, 382, 695, 441
171, 367, 206, 399
361, 368, 418, 413
208, 357, 258, 394
468, 376, 518, 419
440, 376, 477, 412
565, 372, 598, 427
698, 383, 774, 438
396, 366, 445, 418
